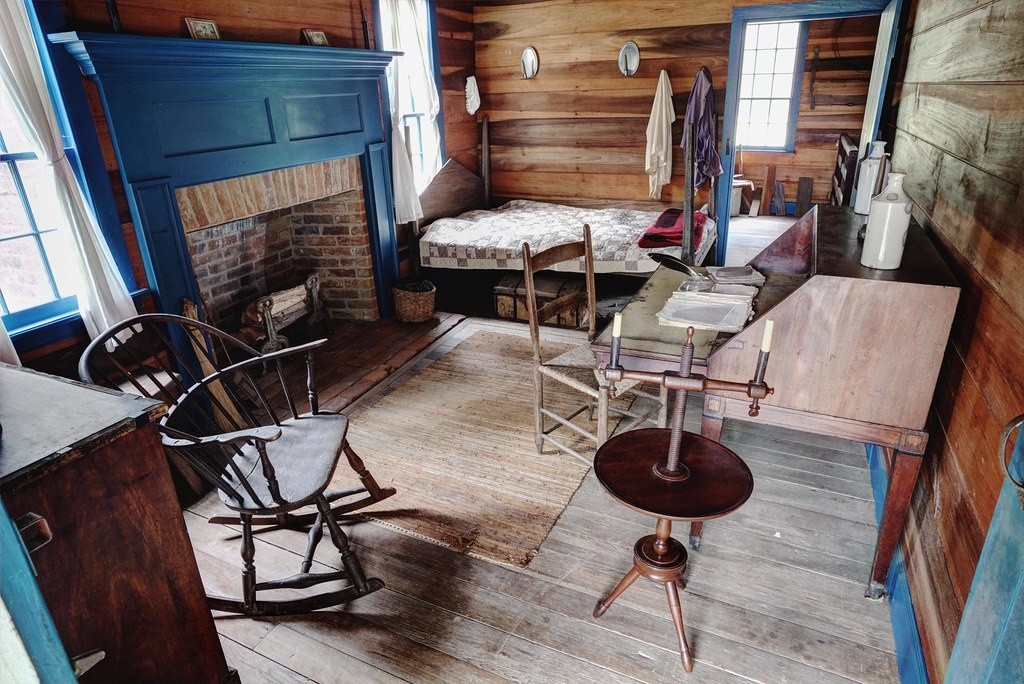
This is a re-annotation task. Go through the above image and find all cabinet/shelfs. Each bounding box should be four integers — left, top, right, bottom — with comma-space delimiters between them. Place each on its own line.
830, 133, 858, 207
0, 362, 242, 684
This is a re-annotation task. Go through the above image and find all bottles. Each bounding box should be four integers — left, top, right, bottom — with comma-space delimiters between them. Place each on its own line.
854, 142, 891, 215
861, 172, 913, 269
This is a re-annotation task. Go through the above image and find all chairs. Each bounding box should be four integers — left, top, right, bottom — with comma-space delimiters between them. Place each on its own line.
521, 224, 667, 471
78, 313, 398, 615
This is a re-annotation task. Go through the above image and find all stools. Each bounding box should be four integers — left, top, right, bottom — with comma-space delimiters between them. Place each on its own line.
100, 364, 204, 496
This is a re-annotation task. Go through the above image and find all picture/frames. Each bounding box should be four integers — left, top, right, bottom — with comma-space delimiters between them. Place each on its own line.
185, 17, 223, 41
303, 29, 331, 47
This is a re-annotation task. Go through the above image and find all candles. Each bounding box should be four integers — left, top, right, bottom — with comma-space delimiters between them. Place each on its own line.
611, 312, 623, 338
761, 319, 774, 352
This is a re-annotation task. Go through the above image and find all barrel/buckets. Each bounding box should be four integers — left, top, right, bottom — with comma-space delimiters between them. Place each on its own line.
730, 186, 743, 216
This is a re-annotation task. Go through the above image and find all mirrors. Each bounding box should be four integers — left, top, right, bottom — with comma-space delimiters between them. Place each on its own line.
617, 41, 640, 76
521, 46, 539, 79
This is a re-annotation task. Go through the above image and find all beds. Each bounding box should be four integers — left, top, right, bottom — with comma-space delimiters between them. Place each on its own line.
417, 157, 718, 269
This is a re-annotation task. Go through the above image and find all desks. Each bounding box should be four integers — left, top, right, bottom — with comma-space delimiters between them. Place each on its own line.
589, 204, 962, 601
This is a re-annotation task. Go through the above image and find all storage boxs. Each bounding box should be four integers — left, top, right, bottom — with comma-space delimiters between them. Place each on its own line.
493, 272, 590, 328
730, 187, 742, 217
